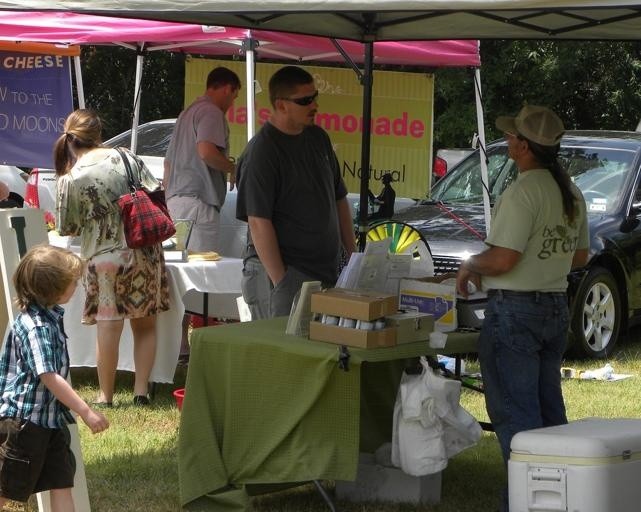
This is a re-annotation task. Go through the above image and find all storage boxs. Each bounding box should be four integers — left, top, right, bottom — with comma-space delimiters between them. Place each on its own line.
336, 453, 442, 505
508, 418, 641, 512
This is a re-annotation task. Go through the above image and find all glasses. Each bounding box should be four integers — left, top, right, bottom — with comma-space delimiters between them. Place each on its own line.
275, 90, 318, 105
503, 130, 517, 141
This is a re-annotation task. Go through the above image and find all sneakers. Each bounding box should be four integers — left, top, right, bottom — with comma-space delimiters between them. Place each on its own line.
92, 403, 111, 409
134, 396, 149, 406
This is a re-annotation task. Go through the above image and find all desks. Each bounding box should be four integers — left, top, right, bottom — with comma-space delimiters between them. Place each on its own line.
57, 252, 244, 401
192, 316, 495, 512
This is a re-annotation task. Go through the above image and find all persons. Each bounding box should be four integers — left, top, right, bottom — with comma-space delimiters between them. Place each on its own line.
163, 67, 241, 255
55, 110, 168, 408
1, 245, 108, 512
456, 104, 590, 464
237, 66, 358, 322
372, 173, 396, 218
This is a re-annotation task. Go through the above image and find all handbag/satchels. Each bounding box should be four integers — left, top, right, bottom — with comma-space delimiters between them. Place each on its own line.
116, 190, 176, 248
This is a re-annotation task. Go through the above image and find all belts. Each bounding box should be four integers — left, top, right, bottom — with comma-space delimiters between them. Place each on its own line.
488, 288, 567, 296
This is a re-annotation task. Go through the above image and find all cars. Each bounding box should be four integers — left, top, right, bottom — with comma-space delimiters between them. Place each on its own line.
434, 146, 477, 182
370, 130, 641, 359
24, 118, 419, 259
0, 164, 29, 209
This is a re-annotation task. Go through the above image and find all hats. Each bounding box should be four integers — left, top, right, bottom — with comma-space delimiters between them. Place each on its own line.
495, 105, 565, 146
